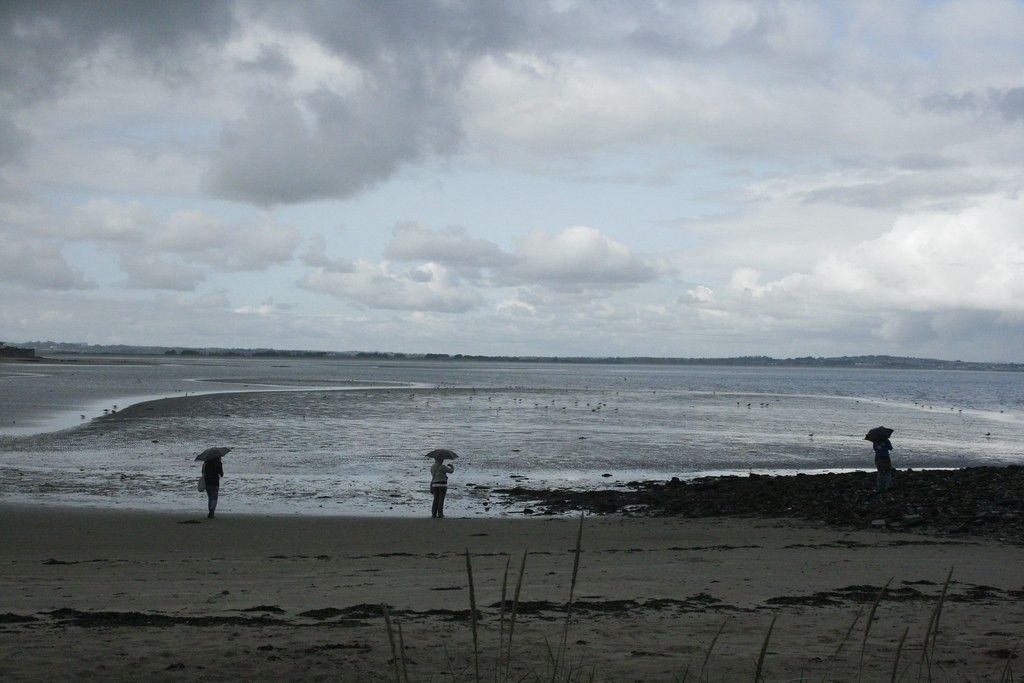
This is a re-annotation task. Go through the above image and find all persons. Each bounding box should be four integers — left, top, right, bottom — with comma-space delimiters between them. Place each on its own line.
873, 440, 893, 489
201, 458, 224, 518
431, 458, 455, 517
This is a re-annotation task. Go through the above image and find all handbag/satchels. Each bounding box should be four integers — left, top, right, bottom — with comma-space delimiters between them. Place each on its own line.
197, 476, 206, 492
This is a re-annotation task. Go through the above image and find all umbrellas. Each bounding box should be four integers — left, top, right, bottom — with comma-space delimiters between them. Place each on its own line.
426, 449, 459, 460
194, 447, 231, 461
865, 426, 895, 444
